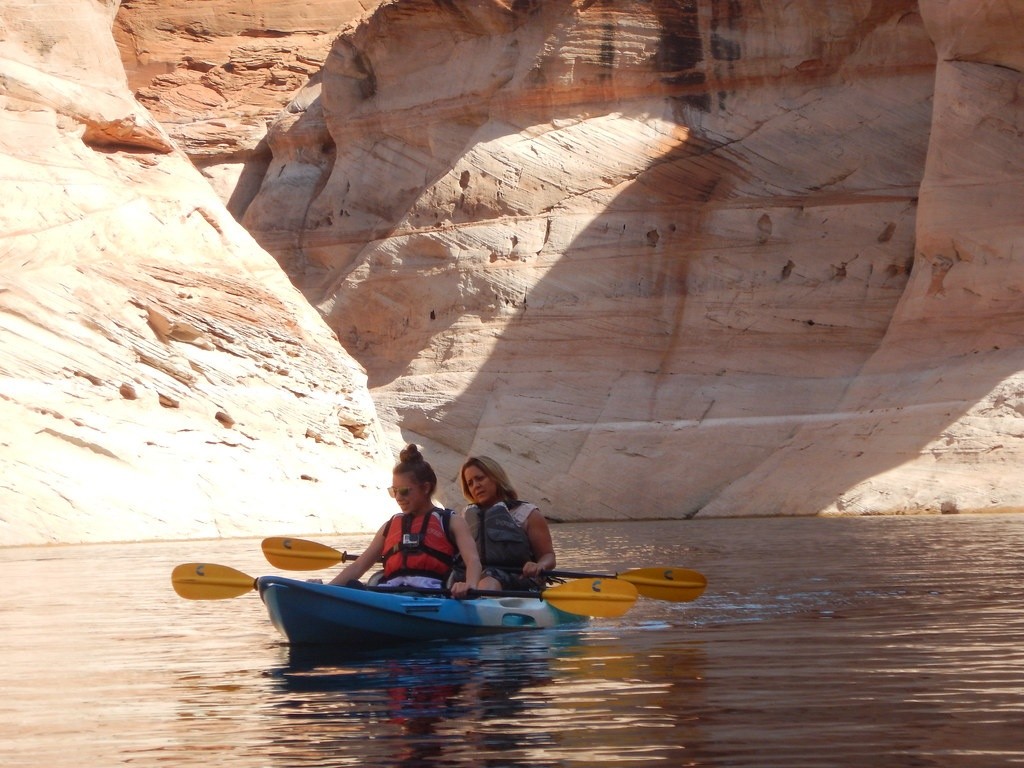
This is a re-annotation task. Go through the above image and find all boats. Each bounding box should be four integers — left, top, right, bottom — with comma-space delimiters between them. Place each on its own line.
255, 575, 592, 646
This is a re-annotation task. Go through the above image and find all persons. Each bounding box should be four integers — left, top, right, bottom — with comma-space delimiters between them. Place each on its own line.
305, 442, 484, 599
446, 454, 557, 593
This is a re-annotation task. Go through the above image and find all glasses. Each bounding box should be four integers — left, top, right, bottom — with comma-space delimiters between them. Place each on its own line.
388, 487, 413, 497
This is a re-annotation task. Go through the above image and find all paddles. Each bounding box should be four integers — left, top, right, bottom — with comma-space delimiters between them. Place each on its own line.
170, 563, 638, 620
259, 535, 708, 600
